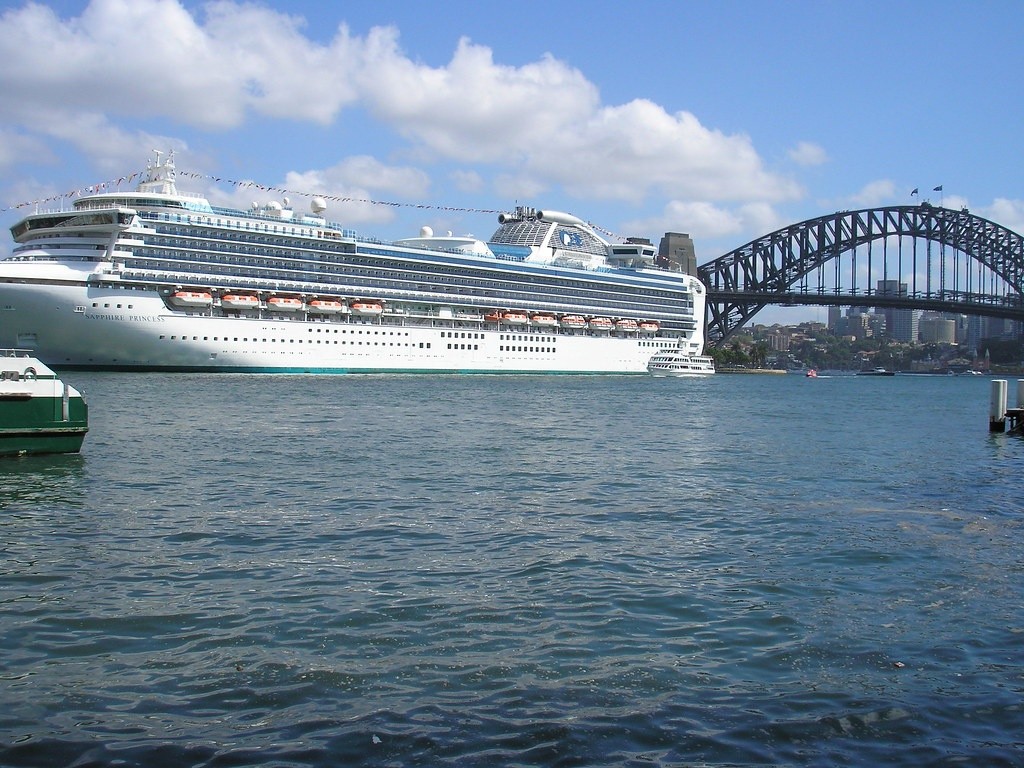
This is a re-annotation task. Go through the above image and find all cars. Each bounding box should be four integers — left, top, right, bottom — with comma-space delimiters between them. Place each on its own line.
873, 367, 885, 373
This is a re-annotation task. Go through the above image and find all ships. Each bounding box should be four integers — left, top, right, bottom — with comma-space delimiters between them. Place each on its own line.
1, 148, 707, 379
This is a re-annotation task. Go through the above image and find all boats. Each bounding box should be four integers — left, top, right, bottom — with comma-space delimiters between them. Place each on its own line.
308, 300, 343, 315
168, 292, 213, 308
220, 293, 261, 310
531, 315, 554, 327
265, 298, 302, 311
0, 346, 90, 457
589, 318, 613, 330
645, 347, 715, 378
502, 313, 527, 325
349, 303, 383, 316
806, 368, 818, 378
561, 315, 585, 329
640, 324, 658, 332
616, 318, 639, 332
